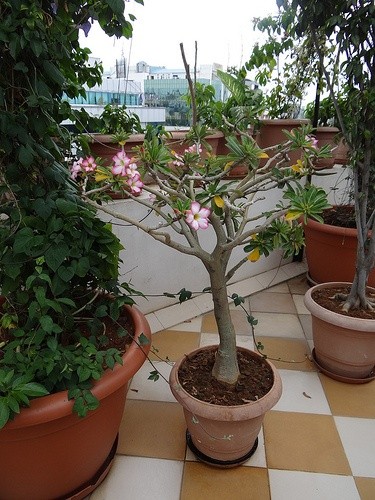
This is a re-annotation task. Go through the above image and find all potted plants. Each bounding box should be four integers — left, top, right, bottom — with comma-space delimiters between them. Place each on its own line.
301, 82, 375, 385
79, 98, 149, 200
157, 65, 350, 182
0, 0, 151, 499
305, 95, 340, 169
241, 1, 375, 386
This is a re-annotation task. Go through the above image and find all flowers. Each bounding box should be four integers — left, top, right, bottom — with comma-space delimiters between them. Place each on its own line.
71, 66, 334, 382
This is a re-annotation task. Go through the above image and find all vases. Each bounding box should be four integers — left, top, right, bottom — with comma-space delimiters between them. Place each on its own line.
168, 344, 283, 469
